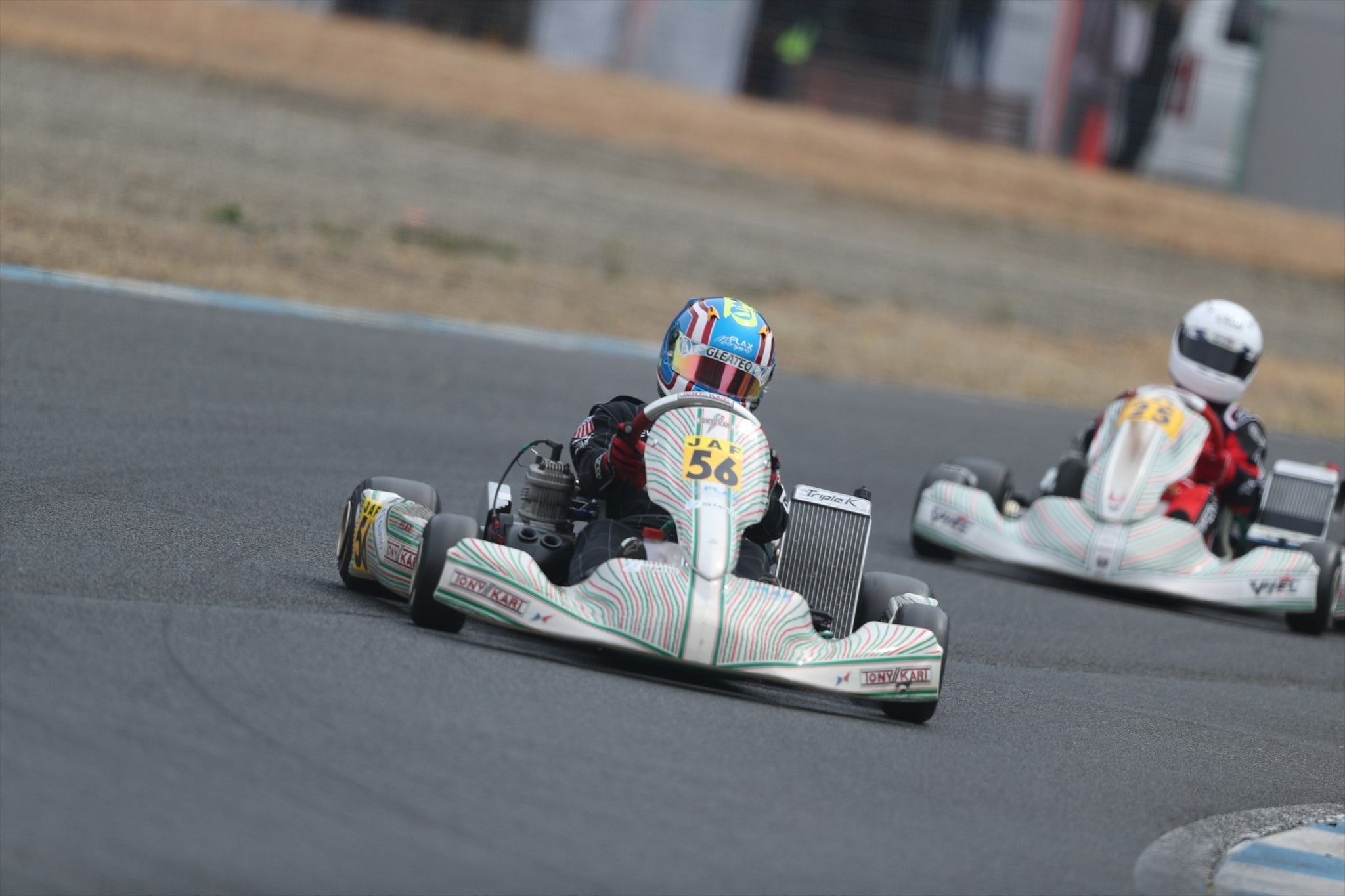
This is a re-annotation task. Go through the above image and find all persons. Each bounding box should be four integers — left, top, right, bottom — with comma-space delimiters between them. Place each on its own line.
572, 297, 835, 641
1001, 298, 1267, 566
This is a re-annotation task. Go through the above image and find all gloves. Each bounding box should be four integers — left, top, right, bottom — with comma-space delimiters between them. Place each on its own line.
606, 421, 646, 474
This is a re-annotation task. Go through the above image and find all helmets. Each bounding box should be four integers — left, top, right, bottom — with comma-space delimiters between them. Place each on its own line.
1169, 298, 1263, 404
656, 297, 774, 413
